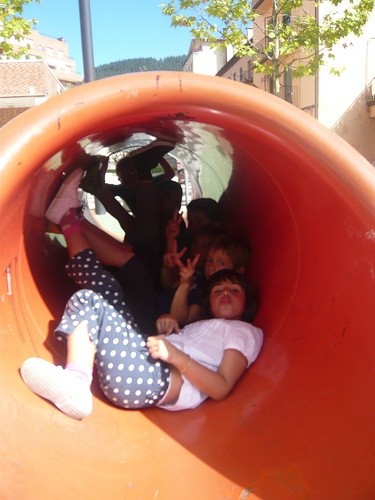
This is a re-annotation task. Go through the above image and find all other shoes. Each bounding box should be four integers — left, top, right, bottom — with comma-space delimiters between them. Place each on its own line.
99, 156, 109, 184
43, 200, 85, 234
80, 159, 101, 194
129, 139, 176, 170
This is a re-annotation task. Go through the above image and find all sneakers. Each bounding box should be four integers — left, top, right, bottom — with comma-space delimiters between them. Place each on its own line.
20, 357, 94, 421
44, 168, 86, 224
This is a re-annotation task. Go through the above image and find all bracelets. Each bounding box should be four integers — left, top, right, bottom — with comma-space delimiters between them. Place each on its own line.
178, 354, 192, 374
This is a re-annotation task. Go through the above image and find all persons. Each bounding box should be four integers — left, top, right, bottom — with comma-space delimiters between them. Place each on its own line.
18, 168, 263, 422
44, 138, 259, 334
